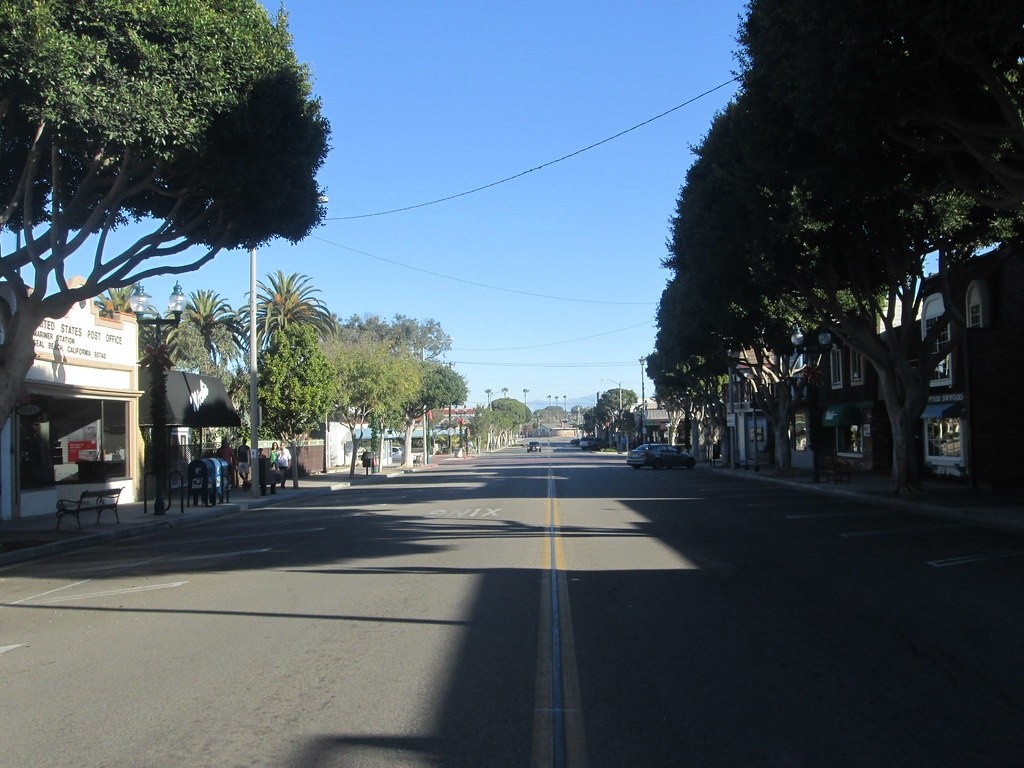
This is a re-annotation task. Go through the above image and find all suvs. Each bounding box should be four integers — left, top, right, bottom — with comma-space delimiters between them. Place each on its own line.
579, 436, 610, 451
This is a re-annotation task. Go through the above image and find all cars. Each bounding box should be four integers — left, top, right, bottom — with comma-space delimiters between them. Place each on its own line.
392, 446, 416, 461
526, 441, 543, 452
626, 442, 696, 470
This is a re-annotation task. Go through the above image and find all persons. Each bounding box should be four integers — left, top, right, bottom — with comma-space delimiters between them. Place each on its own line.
258, 448, 266, 458
217, 438, 237, 465
236, 438, 251, 486
276, 441, 291, 469
269, 442, 279, 463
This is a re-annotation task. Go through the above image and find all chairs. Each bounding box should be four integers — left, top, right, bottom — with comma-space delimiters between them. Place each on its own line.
55, 485, 125, 532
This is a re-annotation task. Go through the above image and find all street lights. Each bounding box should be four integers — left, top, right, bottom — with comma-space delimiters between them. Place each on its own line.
639, 356, 647, 445
601, 378, 622, 452
454, 404, 467, 448
485, 388, 493, 404
488, 391, 507, 451
547, 395, 552, 423
792, 325, 832, 483
501, 387, 509, 398
420, 338, 455, 466
725, 348, 741, 469
584, 396, 598, 438
555, 396, 559, 416
522, 389, 530, 405
127, 280, 188, 515
562, 395, 567, 419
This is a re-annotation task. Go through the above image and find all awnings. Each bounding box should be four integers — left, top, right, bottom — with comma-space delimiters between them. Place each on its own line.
822, 402, 861, 427
922, 402, 965, 418
138, 366, 242, 427
